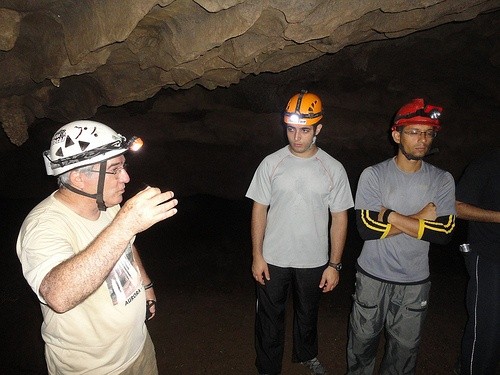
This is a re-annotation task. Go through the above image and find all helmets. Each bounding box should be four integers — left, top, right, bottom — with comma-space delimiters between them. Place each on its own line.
390, 98, 443, 130
283, 89, 323, 127
42, 120, 143, 177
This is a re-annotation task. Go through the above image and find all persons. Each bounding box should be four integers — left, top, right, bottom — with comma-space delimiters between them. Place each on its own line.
16, 120, 178, 375
346, 98, 456, 373
454, 143, 500, 375
243, 90, 356, 374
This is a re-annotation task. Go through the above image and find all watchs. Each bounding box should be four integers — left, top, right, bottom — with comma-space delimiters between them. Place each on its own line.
328, 262, 344, 272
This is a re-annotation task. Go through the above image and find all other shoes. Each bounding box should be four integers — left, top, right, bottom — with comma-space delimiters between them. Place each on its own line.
298, 356, 330, 375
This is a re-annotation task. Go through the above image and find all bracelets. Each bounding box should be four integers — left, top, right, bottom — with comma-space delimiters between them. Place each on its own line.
145, 282, 154, 290
